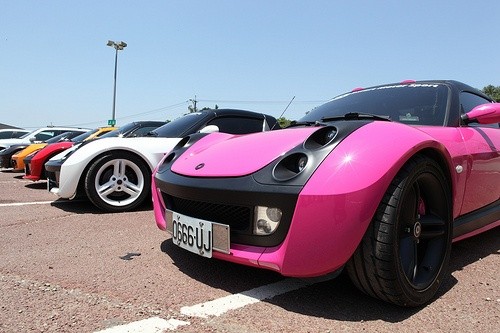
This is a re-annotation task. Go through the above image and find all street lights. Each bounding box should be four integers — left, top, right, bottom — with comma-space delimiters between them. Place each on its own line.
106, 40, 128, 125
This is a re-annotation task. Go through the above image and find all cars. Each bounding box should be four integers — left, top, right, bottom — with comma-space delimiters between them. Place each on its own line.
0, 126, 117, 183
151, 80, 500, 308
99, 120, 168, 138
44, 108, 282, 215
